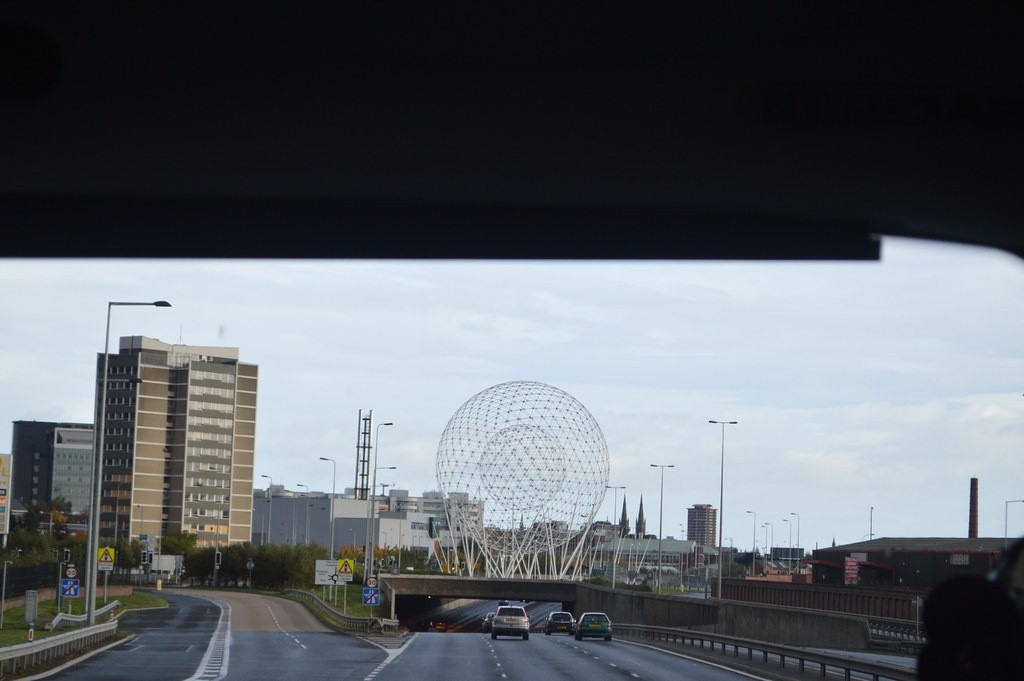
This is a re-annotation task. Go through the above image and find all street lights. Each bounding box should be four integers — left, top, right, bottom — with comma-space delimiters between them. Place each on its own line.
747, 510, 755, 576
212, 485, 223, 587
708, 419, 738, 599
137, 505, 143, 536
262, 475, 273, 546
783, 519, 792, 575
87, 301, 173, 627
765, 522, 774, 575
39, 510, 52, 535
297, 485, 309, 546
870, 507, 874, 541
606, 484, 626, 586
724, 537, 733, 577
320, 457, 337, 603
791, 512, 799, 574
370, 423, 394, 574
650, 464, 675, 593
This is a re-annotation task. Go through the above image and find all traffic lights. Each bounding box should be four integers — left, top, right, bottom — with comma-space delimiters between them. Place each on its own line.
148, 551, 155, 563
63, 549, 70, 563
141, 550, 145, 564
215, 551, 223, 566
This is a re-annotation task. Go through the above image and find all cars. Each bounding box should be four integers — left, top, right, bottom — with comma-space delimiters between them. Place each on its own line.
490, 606, 531, 640
574, 612, 613, 641
543, 611, 576, 635
481, 612, 496, 632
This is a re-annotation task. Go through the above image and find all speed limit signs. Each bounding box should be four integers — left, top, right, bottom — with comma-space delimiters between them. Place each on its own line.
366, 577, 377, 588
65, 567, 77, 579
